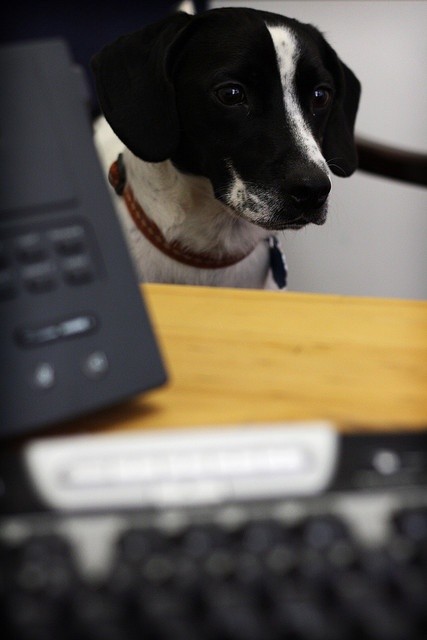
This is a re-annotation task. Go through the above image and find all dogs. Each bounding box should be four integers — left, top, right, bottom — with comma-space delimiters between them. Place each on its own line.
0, 0, 360, 289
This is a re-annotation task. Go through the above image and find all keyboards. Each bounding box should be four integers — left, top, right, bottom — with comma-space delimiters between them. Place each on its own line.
0, 422, 427, 639
